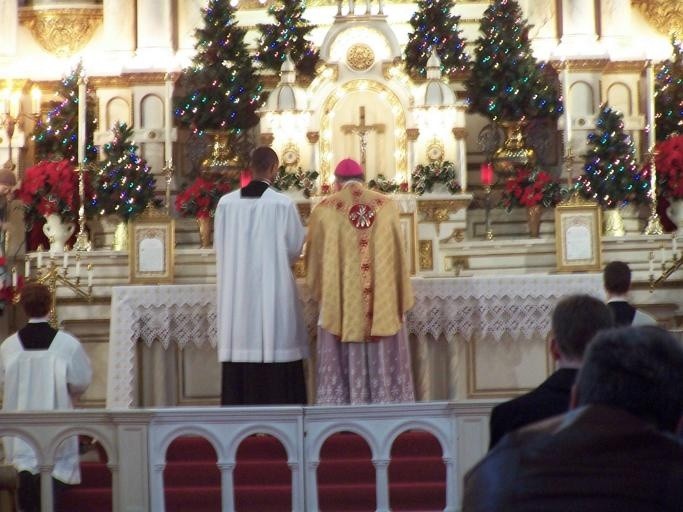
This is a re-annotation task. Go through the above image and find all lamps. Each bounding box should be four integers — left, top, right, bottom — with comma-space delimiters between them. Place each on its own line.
560, 58, 654, 145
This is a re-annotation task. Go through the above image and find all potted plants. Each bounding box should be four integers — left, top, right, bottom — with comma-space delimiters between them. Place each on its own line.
460, 2, 564, 187
89, 120, 165, 253
170, 0, 272, 184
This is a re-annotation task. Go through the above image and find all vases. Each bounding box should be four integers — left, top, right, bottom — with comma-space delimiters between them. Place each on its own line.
431, 182, 447, 195
193, 209, 213, 248
42, 208, 76, 254
668, 195, 683, 230
524, 202, 543, 236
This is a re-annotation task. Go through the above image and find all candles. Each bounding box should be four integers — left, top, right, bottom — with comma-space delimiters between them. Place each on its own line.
77, 79, 174, 163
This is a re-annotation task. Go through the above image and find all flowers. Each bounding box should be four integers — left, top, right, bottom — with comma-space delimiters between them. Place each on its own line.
13, 158, 92, 216
169, 179, 230, 216
412, 162, 461, 194
502, 135, 683, 213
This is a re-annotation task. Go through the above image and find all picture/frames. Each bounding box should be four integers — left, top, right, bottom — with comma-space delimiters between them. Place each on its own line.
126, 202, 173, 283
399, 212, 417, 275
554, 192, 602, 272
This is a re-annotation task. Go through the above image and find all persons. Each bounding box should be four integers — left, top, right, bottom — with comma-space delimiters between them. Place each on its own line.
211, 144, 308, 407
488, 293, 613, 451
602, 260, 658, 327
0, 283, 93, 512
299, 158, 416, 407
460, 325, 683, 511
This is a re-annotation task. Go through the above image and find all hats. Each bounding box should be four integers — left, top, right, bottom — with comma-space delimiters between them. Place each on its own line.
335, 160, 363, 176
